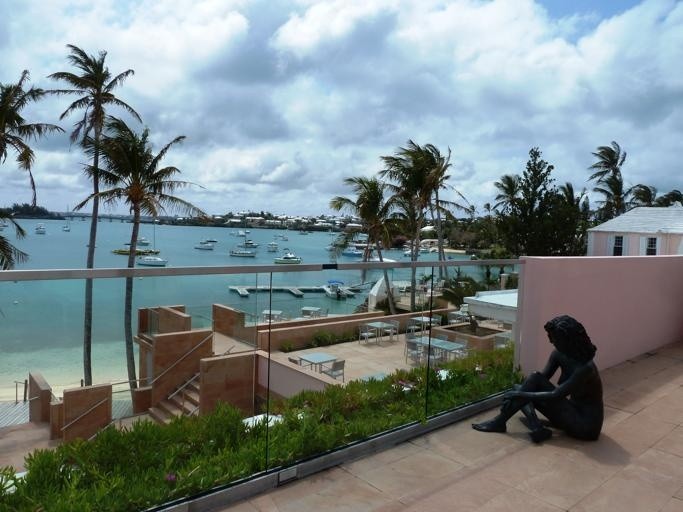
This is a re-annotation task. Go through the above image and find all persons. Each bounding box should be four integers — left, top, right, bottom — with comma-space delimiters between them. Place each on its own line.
472, 316, 604, 444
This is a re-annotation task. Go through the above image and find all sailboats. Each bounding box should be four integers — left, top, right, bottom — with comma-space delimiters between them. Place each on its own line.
228, 221, 258, 259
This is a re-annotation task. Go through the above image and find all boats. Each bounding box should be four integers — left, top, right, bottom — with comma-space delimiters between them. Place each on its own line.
341, 246, 364, 257
63, 223, 71, 232
125, 236, 152, 247
402, 246, 431, 257
272, 248, 302, 264
135, 253, 169, 266
323, 279, 347, 299
192, 226, 295, 255
323, 242, 337, 251
33, 222, 47, 235
328, 231, 336, 237
113, 246, 162, 257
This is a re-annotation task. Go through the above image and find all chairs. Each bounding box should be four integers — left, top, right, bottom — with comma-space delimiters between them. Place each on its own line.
260, 278, 511, 394
